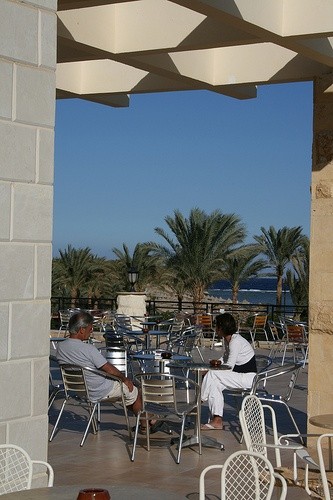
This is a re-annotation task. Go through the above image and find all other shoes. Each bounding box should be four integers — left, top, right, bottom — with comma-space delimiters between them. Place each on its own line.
139, 413, 160, 434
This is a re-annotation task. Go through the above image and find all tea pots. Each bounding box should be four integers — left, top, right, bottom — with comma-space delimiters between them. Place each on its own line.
142, 329, 149, 333
210, 360, 221, 366
161, 352, 174, 358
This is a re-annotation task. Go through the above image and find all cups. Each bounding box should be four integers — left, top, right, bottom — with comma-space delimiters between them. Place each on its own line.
144, 313, 149, 317
220, 309, 224, 314
156, 320, 160, 324
76, 488, 111, 500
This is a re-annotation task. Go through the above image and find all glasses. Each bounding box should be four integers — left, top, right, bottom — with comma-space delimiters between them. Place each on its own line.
90, 327, 94, 332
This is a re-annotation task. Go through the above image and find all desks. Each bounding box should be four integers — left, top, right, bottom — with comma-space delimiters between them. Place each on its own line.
207, 311, 238, 347
131, 315, 164, 330
127, 331, 169, 355
130, 354, 192, 434
309, 414, 333, 430
0, 484, 188, 500
140, 322, 177, 349
282, 321, 308, 361
165, 363, 231, 450
77, 309, 102, 345
49, 338, 66, 389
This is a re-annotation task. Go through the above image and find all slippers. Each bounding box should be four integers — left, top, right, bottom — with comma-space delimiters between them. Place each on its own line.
200, 423, 223, 430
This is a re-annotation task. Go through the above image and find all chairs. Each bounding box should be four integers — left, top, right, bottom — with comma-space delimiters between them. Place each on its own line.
0, 307, 333, 500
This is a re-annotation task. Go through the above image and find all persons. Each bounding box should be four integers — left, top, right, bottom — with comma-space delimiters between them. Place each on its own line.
201, 312, 257, 431
56, 310, 166, 435
